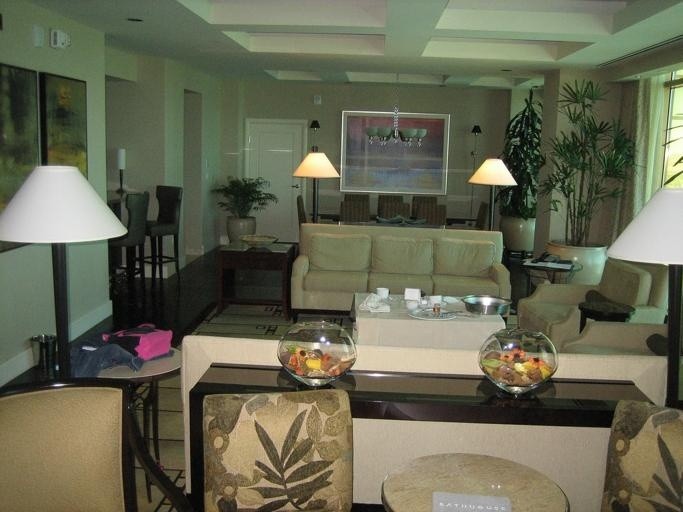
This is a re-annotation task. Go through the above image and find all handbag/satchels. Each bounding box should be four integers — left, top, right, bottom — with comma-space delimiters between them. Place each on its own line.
103, 324, 173, 361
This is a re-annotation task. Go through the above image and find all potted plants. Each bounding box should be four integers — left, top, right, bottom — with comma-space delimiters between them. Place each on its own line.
491, 81, 547, 253
208, 172, 282, 240
535, 76, 642, 285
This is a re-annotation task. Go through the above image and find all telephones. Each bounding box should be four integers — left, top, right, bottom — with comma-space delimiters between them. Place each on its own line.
538, 252, 560, 262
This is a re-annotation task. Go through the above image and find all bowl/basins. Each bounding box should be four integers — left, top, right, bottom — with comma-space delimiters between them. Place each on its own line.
460, 293, 513, 315
236, 235, 278, 249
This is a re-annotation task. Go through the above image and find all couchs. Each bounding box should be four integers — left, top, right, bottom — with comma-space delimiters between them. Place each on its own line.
176, 319, 680, 406
515, 258, 670, 353
285, 220, 512, 325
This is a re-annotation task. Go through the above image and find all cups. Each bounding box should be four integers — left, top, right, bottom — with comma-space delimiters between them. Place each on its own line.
405, 293, 459, 317
376, 287, 389, 299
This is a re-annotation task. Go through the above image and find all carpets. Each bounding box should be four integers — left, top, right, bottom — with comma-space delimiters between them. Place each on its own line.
130, 299, 519, 512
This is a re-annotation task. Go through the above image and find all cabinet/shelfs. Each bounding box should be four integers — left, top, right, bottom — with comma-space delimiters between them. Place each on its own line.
187, 364, 656, 512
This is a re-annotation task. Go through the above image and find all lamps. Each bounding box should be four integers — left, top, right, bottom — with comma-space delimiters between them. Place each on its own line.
290, 151, 340, 221
303, 116, 326, 224
0, 162, 129, 381
114, 148, 127, 194
601, 186, 682, 410
467, 123, 484, 224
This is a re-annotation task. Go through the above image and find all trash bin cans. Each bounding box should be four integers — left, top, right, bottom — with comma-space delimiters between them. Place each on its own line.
31, 334, 57, 369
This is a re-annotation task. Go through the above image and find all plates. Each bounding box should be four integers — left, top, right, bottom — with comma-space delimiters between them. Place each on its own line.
406, 307, 457, 321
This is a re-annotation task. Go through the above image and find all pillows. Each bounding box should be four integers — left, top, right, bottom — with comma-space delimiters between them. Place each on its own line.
371, 236, 433, 276
599, 257, 652, 308
307, 233, 370, 273
432, 238, 495, 278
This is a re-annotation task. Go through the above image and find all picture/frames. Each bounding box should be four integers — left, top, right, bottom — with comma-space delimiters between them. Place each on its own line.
464, 154, 512, 235
338, 108, 452, 198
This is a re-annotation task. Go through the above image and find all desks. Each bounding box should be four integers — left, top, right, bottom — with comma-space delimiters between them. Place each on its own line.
219, 234, 296, 319
375, 450, 577, 512
568, 296, 638, 335
72, 338, 182, 503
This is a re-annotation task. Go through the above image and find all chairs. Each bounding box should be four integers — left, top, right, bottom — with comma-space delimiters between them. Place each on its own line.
594, 398, 682, 512
143, 185, 183, 292
291, 188, 493, 232
0, 377, 135, 512
197, 381, 354, 512
107, 190, 150, 306
